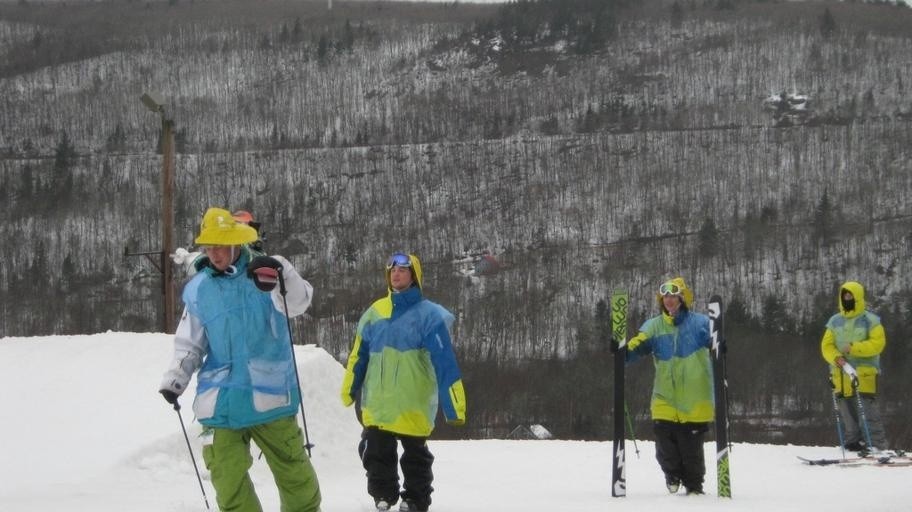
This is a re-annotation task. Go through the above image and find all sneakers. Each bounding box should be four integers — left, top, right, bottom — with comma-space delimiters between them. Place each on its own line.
686, 489, 704, 496
399, 497, 420, 512
665, 474, 680, 493
374, 497, 392, 511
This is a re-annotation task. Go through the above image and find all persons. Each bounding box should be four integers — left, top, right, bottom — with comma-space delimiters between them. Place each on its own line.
160, 207, 323, 512
340, 252, 470, 512
819, 280, 894, 455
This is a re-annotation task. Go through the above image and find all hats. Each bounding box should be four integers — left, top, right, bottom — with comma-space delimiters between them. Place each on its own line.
193, 206, 258, 245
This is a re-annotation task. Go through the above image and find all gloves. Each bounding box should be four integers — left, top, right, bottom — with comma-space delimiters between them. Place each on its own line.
246, 254, 282, 292
160, 388, 178, 404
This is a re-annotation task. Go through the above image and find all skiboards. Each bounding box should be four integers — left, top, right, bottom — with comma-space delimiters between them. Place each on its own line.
796, 450, 912, 467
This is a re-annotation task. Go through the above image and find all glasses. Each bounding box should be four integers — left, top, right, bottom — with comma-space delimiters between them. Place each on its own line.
658, 282, 681, 296
385, 254, 410, 270
